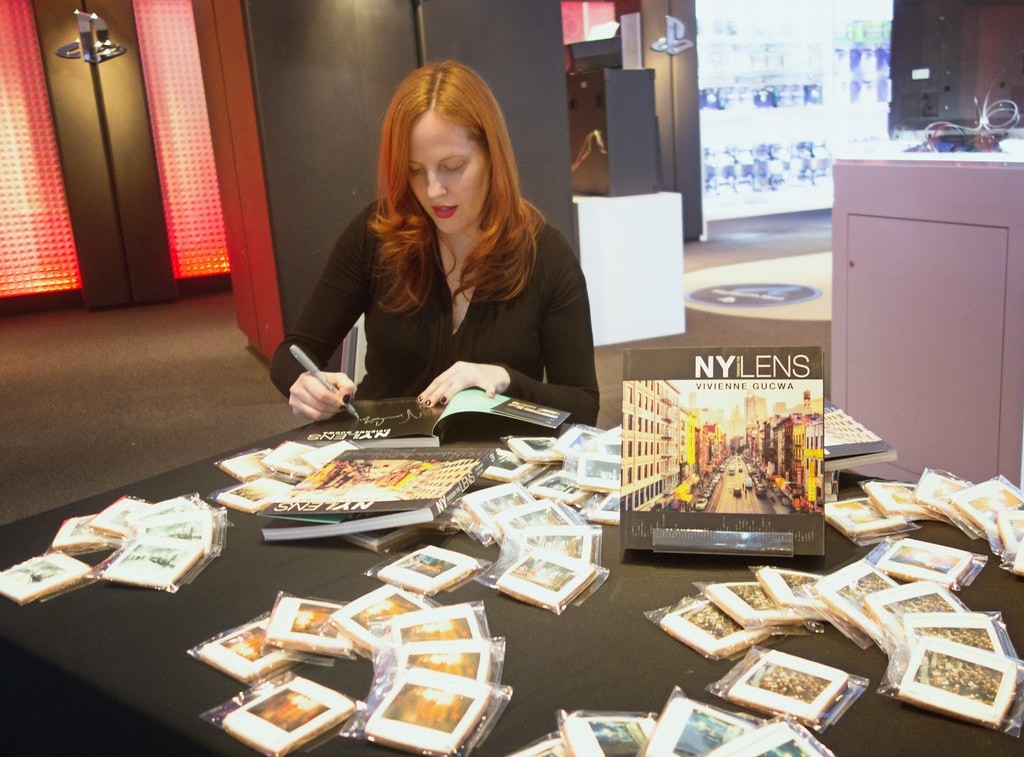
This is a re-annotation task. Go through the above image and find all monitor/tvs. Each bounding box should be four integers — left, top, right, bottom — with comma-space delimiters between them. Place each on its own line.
887, 0, 1024, 154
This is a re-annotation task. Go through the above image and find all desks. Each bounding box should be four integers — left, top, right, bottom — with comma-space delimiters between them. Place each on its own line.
0, 395, 1024, 757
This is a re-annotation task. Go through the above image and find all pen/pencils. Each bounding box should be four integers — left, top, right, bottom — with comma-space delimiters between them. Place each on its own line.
289, 344, 360, 420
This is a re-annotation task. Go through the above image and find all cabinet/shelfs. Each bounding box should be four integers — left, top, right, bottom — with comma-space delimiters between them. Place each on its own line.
831, 136, 1024, 500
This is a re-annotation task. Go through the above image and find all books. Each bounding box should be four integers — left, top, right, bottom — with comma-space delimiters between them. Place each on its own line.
256, 446, 495, 543
824, 391, 898, 503
292, 387, 572, 447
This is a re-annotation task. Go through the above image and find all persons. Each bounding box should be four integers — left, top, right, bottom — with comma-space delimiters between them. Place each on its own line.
270, 59, 602, 437
